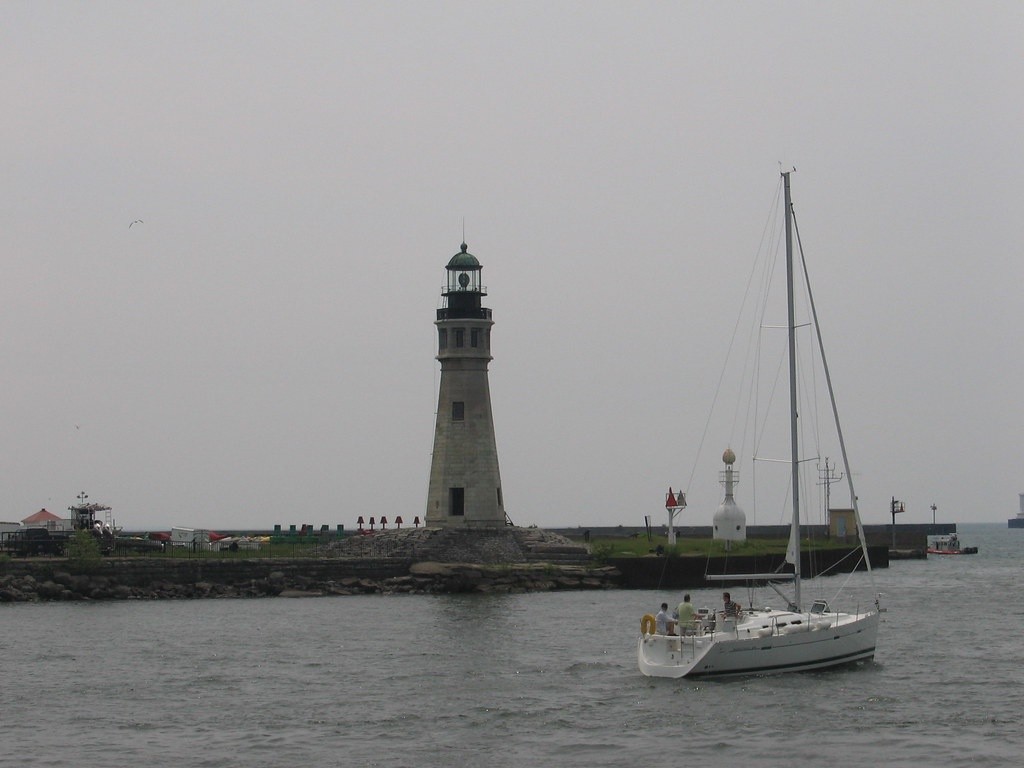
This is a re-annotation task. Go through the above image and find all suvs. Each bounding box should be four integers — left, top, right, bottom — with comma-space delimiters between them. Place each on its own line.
11, 526, 50, 557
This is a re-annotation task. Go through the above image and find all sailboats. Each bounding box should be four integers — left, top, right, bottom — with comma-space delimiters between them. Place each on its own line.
635, 167, 888, 681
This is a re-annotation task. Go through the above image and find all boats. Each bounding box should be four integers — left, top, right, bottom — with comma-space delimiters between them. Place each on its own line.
925, 534, 980, 554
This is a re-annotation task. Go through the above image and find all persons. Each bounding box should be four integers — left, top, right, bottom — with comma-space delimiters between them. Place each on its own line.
655, 603, 678, 636
679, 594, 706, 636
723, 592, 741, 632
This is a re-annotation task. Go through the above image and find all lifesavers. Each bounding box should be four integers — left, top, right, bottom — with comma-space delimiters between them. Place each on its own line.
641, 614, 656, 635
